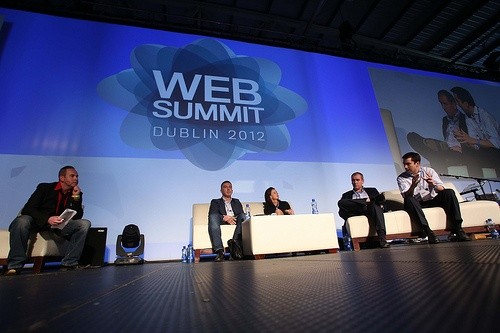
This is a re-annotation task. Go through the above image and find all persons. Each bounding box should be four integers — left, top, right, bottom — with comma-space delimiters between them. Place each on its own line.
396, 151, 472, 244
262, 187, 295, 217
407, 87, 500, 183
338, 171, 390, 248
207, 180, 246, 262
4, 165, 91, 273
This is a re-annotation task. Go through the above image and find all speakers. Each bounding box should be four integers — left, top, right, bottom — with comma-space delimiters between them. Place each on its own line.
78, 227, 107, 267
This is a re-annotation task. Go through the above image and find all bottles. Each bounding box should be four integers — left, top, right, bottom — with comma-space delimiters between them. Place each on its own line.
312, 198, 318, 214
487, 218, 500, 238
245, 204, 250, 216
182, 246, 187, 262
187, 244, 194, 263
345, 231, 352, 251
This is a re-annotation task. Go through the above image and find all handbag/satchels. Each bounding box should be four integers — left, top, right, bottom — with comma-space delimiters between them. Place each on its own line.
227, 233, 255, 260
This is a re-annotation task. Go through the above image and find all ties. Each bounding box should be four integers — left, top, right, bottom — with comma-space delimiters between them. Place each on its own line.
56, 190, 70, 216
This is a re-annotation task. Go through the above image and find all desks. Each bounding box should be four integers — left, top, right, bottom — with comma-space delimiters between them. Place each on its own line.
242, 214, 340, 261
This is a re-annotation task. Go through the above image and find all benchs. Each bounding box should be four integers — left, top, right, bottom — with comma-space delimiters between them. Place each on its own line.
346, 183, 500, 250
191, 202, 265, 263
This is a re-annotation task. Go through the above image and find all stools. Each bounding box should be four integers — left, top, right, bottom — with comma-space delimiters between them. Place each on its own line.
0, 228, 64, 274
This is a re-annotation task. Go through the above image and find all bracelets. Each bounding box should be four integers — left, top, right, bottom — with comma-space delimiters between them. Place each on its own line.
72, 195, 80, 198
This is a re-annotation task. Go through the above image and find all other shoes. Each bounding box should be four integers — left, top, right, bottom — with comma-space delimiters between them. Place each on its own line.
7, 268, 16, 274
428, 232, 440, 243
378, 238, 390, 248
229, 254, 234, 260
372, 192, 386, 204
457, 230, 472, 241
61, 264, 90, 270
215, 250, 225, 262
